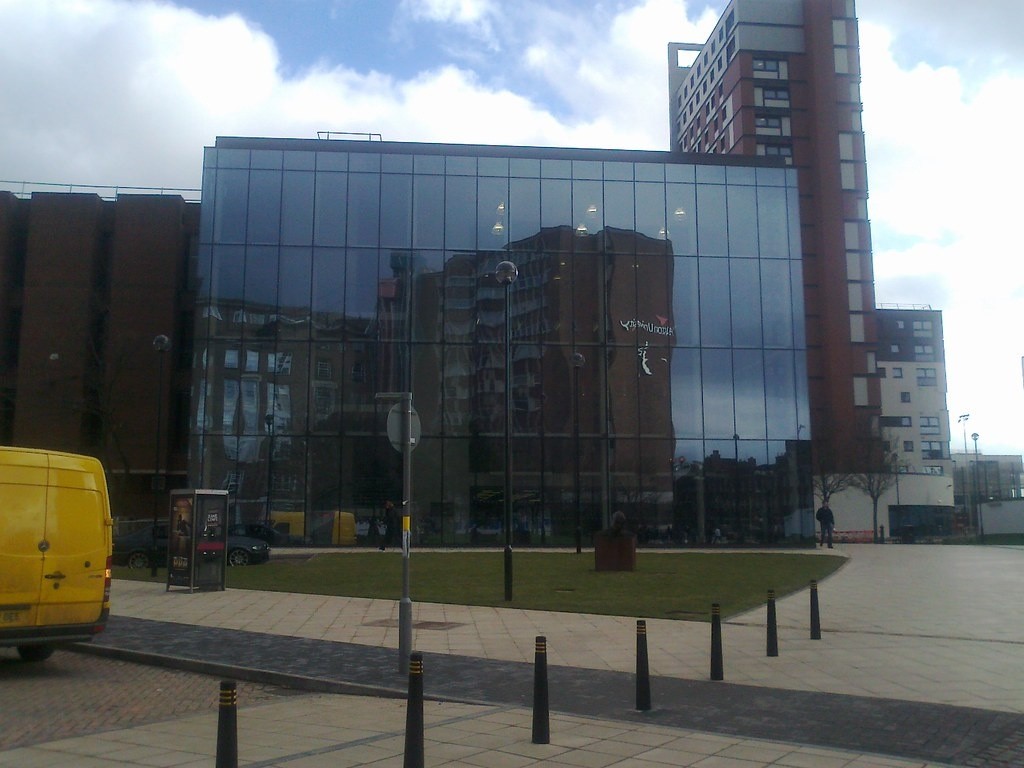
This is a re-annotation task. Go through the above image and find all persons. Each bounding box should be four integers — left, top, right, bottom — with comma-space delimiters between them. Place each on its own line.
379, 501, 402, 551
816, 499, 835, 549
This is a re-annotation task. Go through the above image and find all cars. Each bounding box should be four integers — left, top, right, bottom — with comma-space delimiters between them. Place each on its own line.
113, 524, 269, 569
229, 525, 304, 546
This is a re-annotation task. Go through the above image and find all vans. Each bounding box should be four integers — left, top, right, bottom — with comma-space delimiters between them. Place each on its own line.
0, 445, 113, 662
256, 511, 355, 546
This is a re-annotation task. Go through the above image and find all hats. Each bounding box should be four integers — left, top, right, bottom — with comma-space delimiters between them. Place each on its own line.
822, 499, 828, 503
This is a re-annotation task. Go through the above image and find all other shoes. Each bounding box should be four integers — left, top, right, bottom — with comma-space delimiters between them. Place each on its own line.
820, 542, 824, 546
828, 544, 833, 548
379, 545, 384, 550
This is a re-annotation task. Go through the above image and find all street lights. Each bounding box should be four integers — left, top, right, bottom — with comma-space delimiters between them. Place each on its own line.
375, 391, 413, 672
733, 433, 740, 461
496, 261, 520, 600
891, 453, 900, 505
958, 413, 973, 537
972, 433, 983, 534
151, 335, 172, 575
